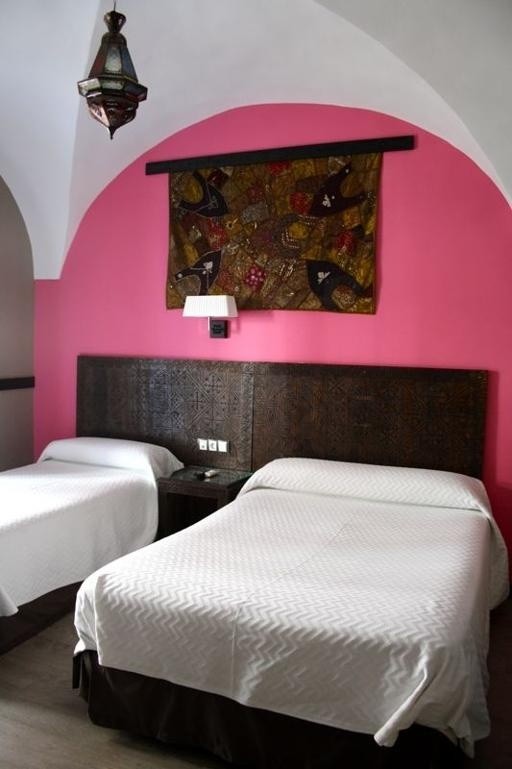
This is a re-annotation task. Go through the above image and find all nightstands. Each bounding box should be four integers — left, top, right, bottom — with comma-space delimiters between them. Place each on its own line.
158, 461, 252, 539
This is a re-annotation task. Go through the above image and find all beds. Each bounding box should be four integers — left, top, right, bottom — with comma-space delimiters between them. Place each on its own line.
0, 434, 179, 659
72, 456, 495, 769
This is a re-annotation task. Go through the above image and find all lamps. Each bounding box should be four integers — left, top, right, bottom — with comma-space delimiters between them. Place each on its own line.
76, 1, 148, 142
181, 294, 239, 339
